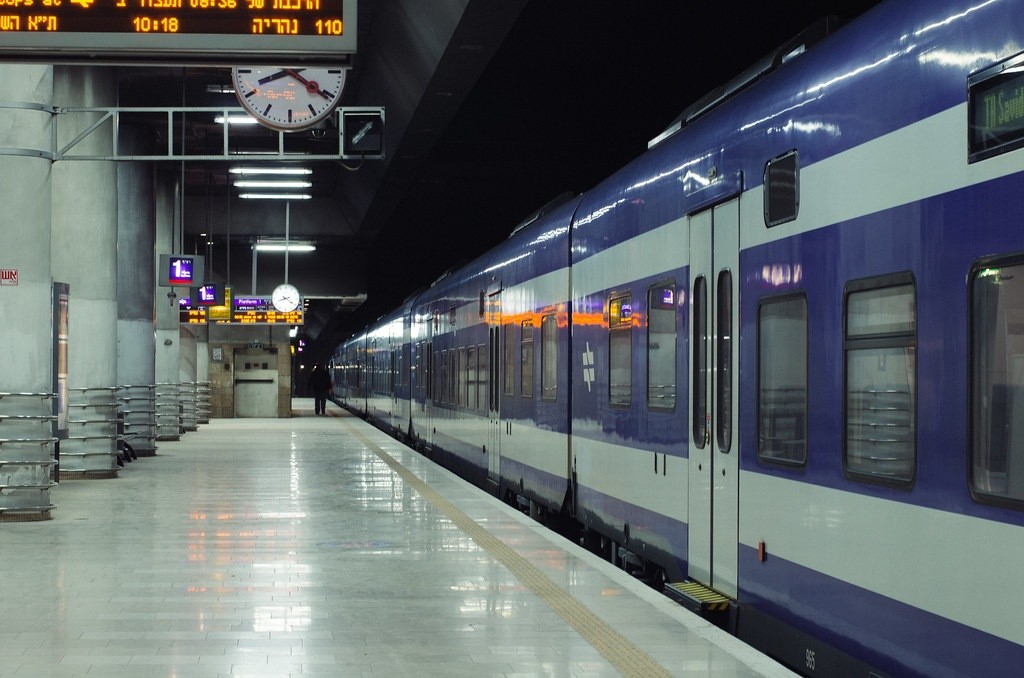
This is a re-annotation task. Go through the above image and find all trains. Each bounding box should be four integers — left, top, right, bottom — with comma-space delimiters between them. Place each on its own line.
320, 0, 1023, 678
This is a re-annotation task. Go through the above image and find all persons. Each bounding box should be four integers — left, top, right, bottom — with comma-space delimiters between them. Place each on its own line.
308, 362, 332, 415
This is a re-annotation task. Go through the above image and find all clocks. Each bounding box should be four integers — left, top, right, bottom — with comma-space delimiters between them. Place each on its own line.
237, 64, 345, 132
272, 284, 300, 312
